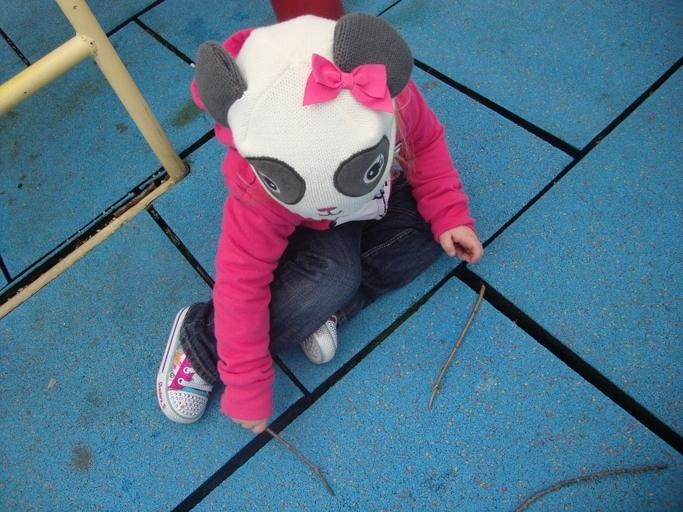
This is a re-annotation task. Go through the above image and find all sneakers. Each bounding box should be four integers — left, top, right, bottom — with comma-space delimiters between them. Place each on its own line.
298, 312, 341, 366
155, 301, 211, 425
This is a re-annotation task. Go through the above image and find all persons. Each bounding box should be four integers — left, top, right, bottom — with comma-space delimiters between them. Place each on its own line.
155, 14, 484, 438
